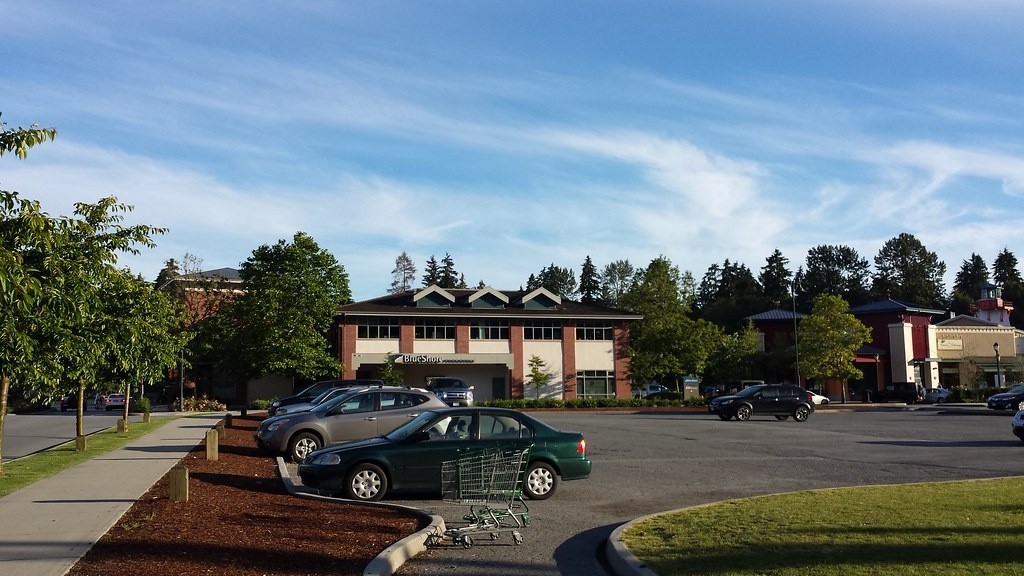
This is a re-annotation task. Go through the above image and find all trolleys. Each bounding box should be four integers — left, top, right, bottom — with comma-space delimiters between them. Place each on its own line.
437, 448, 529, 549
456, 438, 536, 527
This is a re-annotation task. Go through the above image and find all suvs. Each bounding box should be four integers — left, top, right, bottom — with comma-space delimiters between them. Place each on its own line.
274, 384, 428, 416
426, 378, 474, 407
871, 381, 927, 405
267, 379, 385, 418
708, 383, 816, 422
252, 384, 458, 465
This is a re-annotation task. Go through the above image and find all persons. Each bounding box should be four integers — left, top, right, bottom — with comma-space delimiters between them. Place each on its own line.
916, 381, 926, 401
937, 383, 942, 388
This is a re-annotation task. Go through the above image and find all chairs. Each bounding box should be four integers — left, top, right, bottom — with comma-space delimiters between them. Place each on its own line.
449, 419, 466, 437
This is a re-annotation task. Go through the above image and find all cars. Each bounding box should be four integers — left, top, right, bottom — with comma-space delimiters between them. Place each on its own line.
632, 384, 668, 399
104, 393, 133, 411
60, 390, 89, 412
1012, 408, 1024, 442
806, 390, 830, 404
916, 387, 953, 403
295, 406, 592, 502
987, 384, 1024, 410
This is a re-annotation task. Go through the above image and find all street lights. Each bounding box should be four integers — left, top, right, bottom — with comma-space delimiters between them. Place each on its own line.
874, 353, 880, 389
791, 277, 807, 387
993, 343, 1001, 387
178, 334, 187, 413
